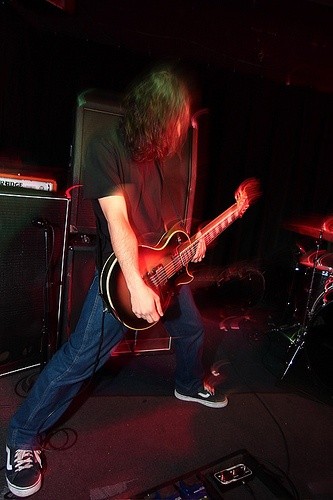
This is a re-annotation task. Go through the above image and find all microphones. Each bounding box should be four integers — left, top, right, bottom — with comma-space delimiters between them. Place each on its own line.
31, 216, 52, 230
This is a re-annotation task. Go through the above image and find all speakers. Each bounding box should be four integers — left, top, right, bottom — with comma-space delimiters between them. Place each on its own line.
0, 185, 70, 377
69, 94, 198, 236
58, 245, 171, 357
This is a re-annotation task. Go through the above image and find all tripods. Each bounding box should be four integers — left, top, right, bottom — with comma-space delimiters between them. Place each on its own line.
249, 232, 333, 381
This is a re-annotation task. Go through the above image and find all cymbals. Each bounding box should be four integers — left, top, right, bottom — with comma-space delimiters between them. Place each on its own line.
292, 216, 333, 242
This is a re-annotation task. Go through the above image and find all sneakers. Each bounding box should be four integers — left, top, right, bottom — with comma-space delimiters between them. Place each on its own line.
174, 380, 229, 408
5, 442, 44, 498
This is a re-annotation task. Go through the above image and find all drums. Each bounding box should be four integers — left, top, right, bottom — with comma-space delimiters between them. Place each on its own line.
309, 285, 333, 322
295, 262, 333, 307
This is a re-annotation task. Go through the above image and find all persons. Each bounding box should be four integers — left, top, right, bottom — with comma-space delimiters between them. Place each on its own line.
4, 71, 228, 497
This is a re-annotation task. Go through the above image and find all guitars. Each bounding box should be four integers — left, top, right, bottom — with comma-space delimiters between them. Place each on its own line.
98, 180, 258, 331
